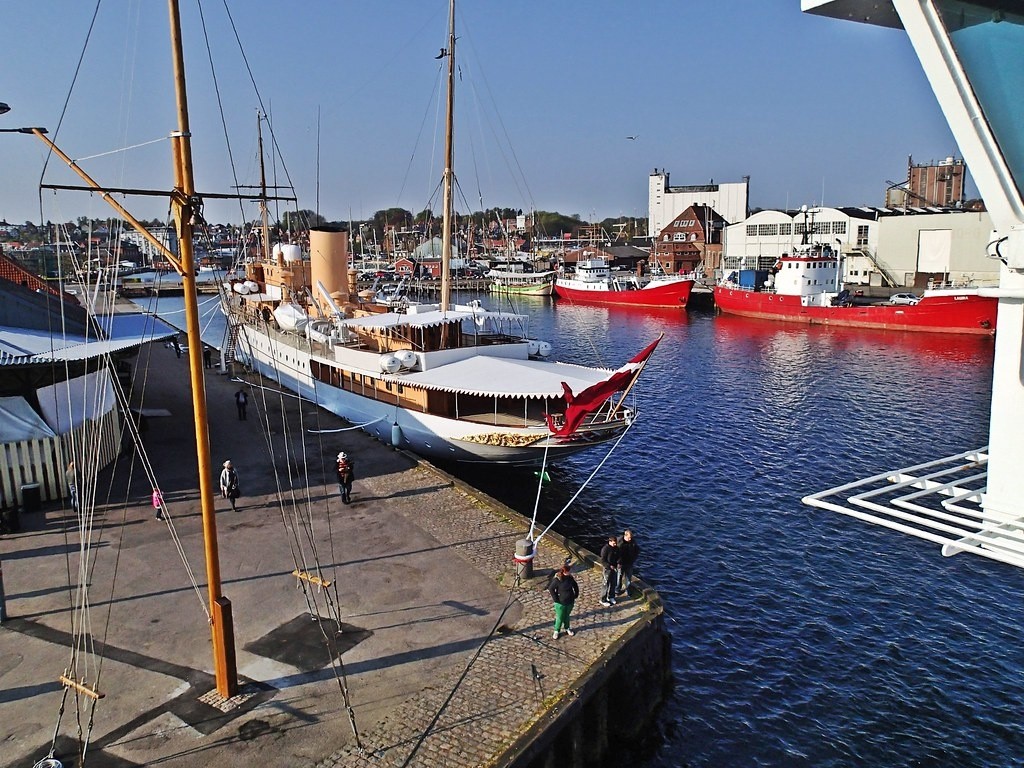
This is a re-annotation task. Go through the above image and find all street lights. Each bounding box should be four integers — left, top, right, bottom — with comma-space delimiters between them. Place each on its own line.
1, 128, 238, 698
83, 259, 103, 308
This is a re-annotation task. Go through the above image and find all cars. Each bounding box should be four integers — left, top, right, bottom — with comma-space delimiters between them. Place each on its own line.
357, 270, 489, 280
889, 293, 920, 306
629, 268, 638, 274
610, 265, 621, 271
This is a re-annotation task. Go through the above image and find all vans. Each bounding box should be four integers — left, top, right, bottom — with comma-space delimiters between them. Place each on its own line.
651, 268, 663, 275
620, 265, 628, 269
565, 266, 575, 272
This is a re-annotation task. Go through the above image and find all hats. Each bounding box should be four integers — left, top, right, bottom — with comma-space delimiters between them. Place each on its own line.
223, 460, 230, 468
204, 346, 208, 348
337, 452, 347, 459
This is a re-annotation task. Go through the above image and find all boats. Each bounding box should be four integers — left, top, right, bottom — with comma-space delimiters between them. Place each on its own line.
554, 209, 695, 309
713, 204, 998, 335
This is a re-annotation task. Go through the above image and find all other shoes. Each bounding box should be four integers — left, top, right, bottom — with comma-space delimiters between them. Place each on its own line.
155, 517, 162, 521
177, 354, 180, 358
625, 588, 633, 596
231, 508, 239, 512
565, 628, 574, 636
615, 591, 621, 596
342, 497, 350, 505
206, 367, 211, 369
239, 418, 248, 421
552, 630, 561, 639
599, 599, 610, 607
609, 597, 616, 604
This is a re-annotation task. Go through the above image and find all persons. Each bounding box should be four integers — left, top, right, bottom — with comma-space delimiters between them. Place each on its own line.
256, 307, 260, 322
153, 487, 163, 521
239, 297, 246, 313
66, 462, 81, 512
173, 336, 181, 358
235, 387, 248, 421
203, 346, 211, 369
549, 565, 579, 640
220, 461, 239, 512
334, 452, 354, 502
262, 306, 272, 325
599, 530, 637, 607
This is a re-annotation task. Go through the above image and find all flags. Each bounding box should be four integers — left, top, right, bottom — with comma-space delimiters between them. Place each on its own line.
543, 337, 658, 436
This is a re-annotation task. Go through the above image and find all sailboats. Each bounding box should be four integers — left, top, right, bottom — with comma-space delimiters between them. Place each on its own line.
219, 1, 643, 465
487, 203, 558, 295
